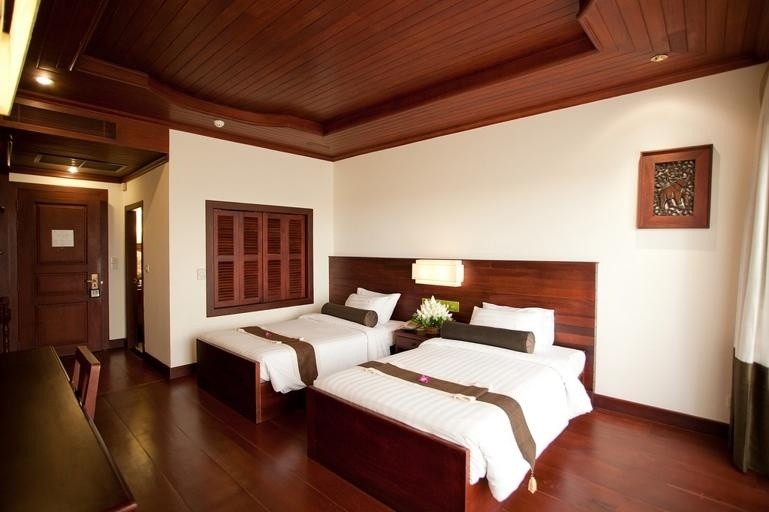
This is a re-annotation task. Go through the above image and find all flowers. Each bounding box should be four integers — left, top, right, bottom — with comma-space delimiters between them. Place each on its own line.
411, 295, 456, 328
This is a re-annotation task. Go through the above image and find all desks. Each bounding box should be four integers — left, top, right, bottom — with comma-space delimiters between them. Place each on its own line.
0, 344, 138, 511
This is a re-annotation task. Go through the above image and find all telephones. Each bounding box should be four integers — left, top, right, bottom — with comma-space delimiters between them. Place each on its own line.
399, 320, 417, 330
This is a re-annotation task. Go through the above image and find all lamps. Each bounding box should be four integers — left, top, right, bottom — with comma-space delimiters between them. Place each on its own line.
411, 259, 465, 288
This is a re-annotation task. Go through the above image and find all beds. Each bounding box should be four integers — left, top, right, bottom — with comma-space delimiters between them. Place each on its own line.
195, 254, 419, 429
306, 258, 598, 512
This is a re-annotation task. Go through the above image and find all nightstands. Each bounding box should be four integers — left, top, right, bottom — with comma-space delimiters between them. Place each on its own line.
393, 328, 439, 352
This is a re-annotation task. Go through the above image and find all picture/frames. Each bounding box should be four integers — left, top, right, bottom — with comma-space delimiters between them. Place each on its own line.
636, 144, 714, 229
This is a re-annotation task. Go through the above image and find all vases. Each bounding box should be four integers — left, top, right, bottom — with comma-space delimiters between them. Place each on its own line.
423, 325, 440, 334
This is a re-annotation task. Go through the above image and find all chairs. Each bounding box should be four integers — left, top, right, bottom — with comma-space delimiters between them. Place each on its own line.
69, 344, 102, 419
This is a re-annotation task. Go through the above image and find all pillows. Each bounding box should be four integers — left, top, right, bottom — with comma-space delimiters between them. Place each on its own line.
356, 287, 401, 316
439, 320, 536, 354
320, 302, 378, 327
480, 300, 555, 345
469, 305, 550, 352
346, 293, 393, 323
